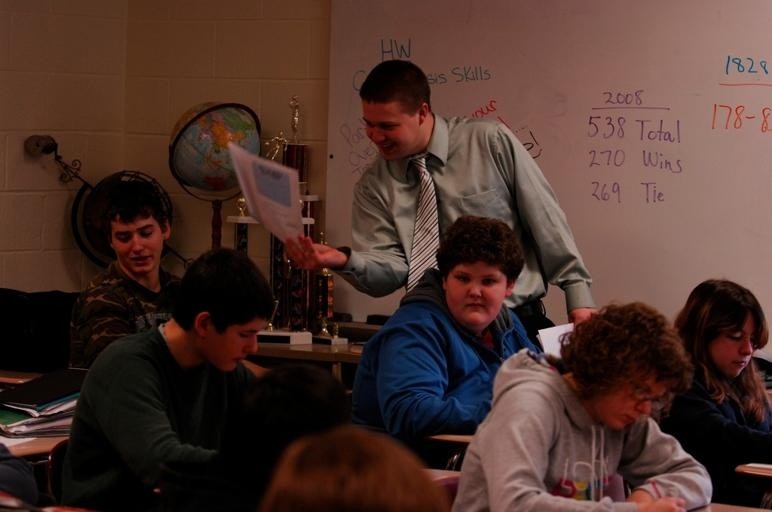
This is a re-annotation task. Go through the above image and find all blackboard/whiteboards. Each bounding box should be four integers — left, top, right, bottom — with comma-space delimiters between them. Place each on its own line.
324, 0, 772, 363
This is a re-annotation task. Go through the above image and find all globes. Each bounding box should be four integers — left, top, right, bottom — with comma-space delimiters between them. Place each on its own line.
167, 101, 261, 249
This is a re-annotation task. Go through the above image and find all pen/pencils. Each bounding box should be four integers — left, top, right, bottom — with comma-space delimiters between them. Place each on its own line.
650, 480, 663, 499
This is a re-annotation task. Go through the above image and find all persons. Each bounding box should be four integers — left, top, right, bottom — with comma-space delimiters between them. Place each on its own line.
260, 427, 450, 512
654, 280, 771, 508
350, 213, 544, 467
0, 443, 38, 505
64, 247, 288, 512
284, 61, 598, 351
451, 300, 713, 512
65, 180, 184, 395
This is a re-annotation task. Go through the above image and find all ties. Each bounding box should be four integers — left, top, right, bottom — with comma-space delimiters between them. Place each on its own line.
406, 155, 441, 294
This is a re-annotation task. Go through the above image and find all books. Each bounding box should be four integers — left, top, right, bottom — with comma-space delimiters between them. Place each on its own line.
0, 372, 81, 438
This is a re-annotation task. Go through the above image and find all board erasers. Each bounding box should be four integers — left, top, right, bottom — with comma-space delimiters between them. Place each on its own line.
366, 314, 392, 325
328, 312, 352, 322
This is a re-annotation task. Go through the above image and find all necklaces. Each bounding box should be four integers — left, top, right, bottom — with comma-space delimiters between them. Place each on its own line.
160, 323, 170, 348
